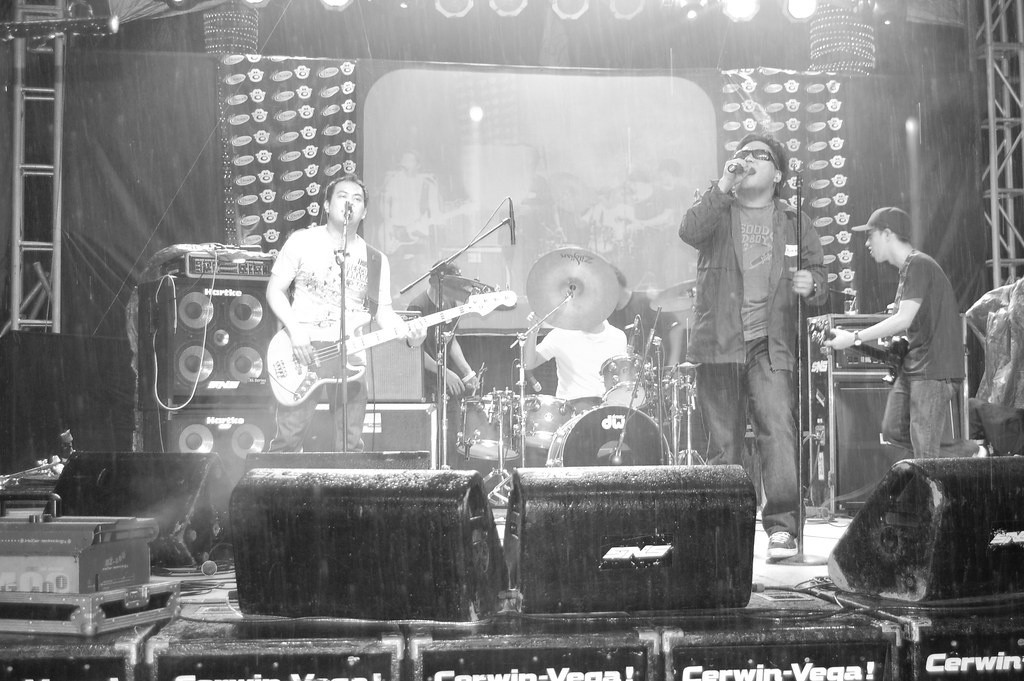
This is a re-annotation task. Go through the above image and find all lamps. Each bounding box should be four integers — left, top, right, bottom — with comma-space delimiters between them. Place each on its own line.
721, 0, 819, 23
434, 0, 645, 21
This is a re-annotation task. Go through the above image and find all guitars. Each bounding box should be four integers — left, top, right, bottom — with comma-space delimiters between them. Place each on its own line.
808, 318, 910, 385
267, 291, 519, 407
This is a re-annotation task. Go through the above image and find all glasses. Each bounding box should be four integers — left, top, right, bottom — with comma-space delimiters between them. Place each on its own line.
732, 149, 778, 173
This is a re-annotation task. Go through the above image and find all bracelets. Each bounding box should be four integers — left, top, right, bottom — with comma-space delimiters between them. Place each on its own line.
528, 326, 540, 334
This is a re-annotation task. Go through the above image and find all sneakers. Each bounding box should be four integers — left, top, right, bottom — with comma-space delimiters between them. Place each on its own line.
767, 532, 798, 559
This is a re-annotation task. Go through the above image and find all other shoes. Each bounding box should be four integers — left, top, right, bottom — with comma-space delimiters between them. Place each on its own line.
972, 445, 987, 458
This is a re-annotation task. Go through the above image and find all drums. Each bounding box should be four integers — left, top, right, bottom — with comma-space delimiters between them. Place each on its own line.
456, 390, 521, 462
569, 397, 601, 411
598, 354, 655, 410
521, 394, 573, 450
545, 406, 671, 467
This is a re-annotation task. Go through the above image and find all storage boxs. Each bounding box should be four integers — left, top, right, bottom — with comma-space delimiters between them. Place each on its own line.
296, 403, 437, 471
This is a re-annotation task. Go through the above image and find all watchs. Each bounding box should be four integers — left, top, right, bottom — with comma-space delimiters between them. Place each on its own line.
802, 279, 817, 298
854, 330, 861, 347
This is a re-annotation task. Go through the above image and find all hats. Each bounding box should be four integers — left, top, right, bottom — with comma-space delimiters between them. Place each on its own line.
853, 208, 909, 240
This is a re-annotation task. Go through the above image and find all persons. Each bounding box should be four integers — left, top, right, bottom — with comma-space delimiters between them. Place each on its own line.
266, 174, 429, 454
823, 206, 988, 462
678, 132, 829, 560
522, 312, 628, 402
406, 259, 480, 470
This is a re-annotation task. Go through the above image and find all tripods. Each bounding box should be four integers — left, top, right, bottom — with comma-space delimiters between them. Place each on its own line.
678, 369, 707, 466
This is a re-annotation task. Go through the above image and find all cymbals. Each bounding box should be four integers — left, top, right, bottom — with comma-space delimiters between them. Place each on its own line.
648, 279, 696, 314
524, 246, 623, 331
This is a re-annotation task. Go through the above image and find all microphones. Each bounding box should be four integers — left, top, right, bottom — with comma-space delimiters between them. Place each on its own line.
524, 367, 542, 392
344, 202, 354, 219
508, 199, 515, 245
727, 163, 745, 174
610, 434, 625, 466
201, 560, 218, 576
626, 316, 640, 355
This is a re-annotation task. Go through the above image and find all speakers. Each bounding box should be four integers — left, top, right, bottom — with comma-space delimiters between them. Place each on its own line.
43, 275, 757, 623
834, 380, 960, 511
826, 456, 1024, 603
